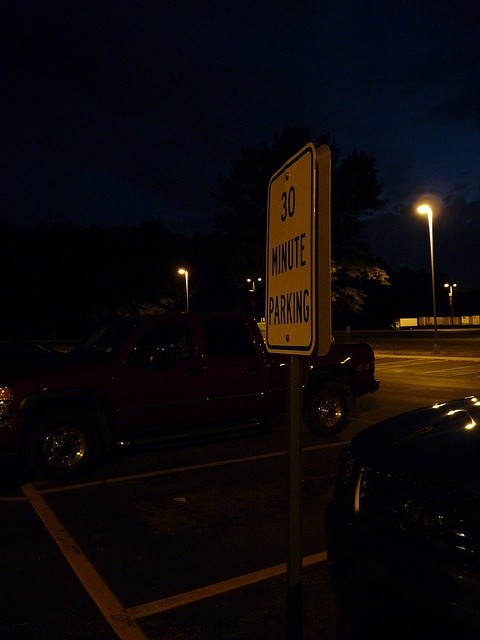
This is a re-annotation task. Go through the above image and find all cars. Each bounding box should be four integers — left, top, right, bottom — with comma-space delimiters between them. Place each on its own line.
323, 394, 479, 637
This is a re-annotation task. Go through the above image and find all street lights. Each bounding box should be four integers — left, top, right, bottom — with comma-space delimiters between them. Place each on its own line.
171, 267, 199, 314
239, 276, 265, 319
443, 284, 464, 338
408, 192, 450, 348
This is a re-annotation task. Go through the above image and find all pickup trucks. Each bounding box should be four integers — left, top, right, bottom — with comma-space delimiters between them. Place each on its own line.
1, 310, 379, 482
